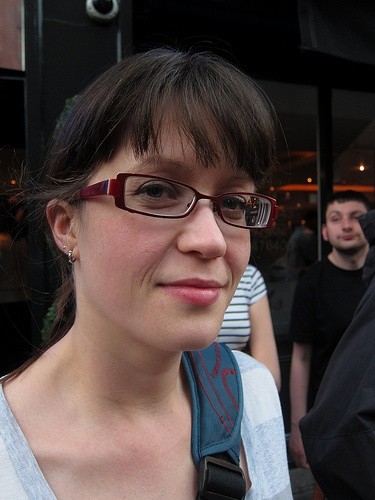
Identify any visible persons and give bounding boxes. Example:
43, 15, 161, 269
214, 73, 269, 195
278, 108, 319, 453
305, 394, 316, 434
0, 49, 294, 500
1, 189, 375, 500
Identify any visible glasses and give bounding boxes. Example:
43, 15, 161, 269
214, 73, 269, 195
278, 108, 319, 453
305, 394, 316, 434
70, 173, 277, 228
